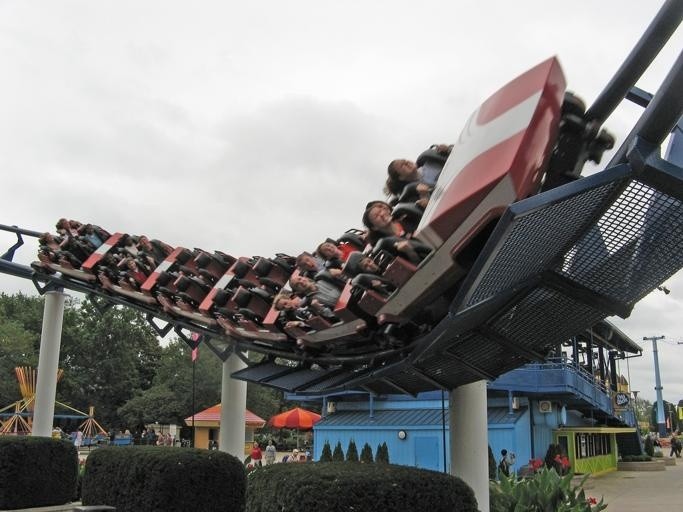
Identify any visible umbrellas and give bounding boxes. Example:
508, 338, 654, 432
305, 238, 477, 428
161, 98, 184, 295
269, 407, 322, 449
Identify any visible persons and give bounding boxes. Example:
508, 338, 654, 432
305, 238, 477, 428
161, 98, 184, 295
73, 427, 171, 451
545, 443, 570, 474
252, 442, 262, 467
274, 143, 451, 331
500, 450, 516, 476
654, 433, 682, 457
38, 218, 170, 292
286, 449, 298, 463
264, 439, 276, 466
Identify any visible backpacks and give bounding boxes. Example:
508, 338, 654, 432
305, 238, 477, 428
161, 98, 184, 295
498, 457, 509, 477
282, 455, 289, 462
